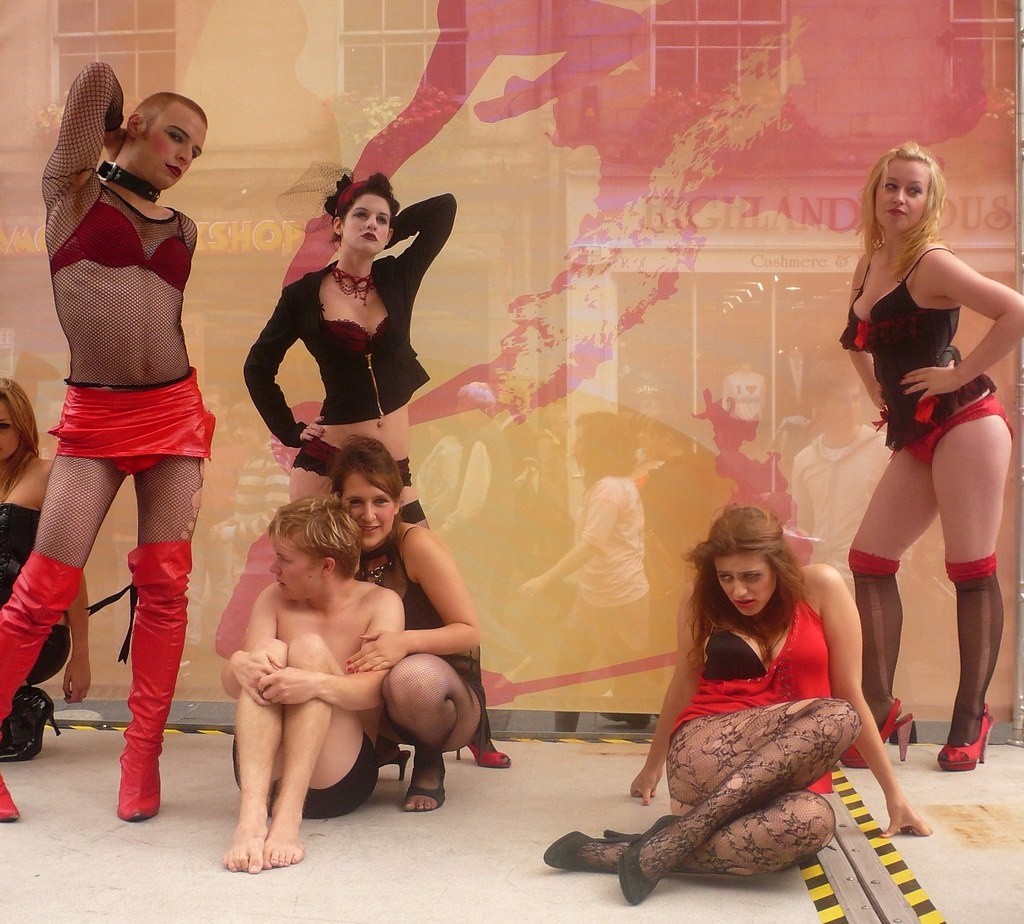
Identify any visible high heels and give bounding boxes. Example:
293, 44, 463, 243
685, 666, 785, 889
0, 686, 61, 762
937, 704, 992, 771
378, 749, 411, 781
841, 698, 913, 768
618, 815, 684, 905
403, 755, 445, 812
455, 740, 512, 768
544, 829, 641, 880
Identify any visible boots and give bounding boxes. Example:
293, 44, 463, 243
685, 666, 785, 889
117, 541, 193, 821
0, 550, 84, 822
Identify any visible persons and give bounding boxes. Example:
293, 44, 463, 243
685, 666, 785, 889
837, 139, 1024, 773
765, 347, 818, 432
119, 362, 919, 734
223, 431, 483, 812
224, 494, 405, 875
0, 61, 219, 822
245, 162, 512, 767
0, 378, 92, 762
723, 359, 766, 424
544, 507, 933, 905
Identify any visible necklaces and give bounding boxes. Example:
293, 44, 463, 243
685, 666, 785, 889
97, 160, 161, 203
330, 265, 377, 307
360, 560, 396, 585
